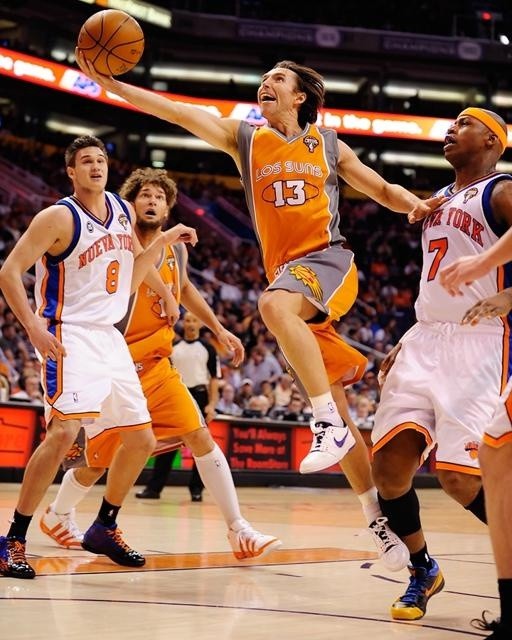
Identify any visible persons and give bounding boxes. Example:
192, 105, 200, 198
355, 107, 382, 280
469, 373, 512, 640
0, 136, 158, 579
371, 106, 512, 640
135, 311, 222, 502
73, 44, 450, 571
39, 165, 284, 560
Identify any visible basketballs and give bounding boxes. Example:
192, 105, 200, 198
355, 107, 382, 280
78, 10, 144, 76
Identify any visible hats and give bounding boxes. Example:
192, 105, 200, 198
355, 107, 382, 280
240, 378, 253, 387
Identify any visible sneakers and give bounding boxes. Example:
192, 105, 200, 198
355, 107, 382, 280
297, 415, 358, 476
224, 518, 284, 564
39, 504, 85, 551
135, 488, 161, 500
191, 491, 203, 502
470, 609, 512, 640
389, 557, 446, 622
0, 534, 36, 580
366, 516, 411, 572
81, 520, 145, 568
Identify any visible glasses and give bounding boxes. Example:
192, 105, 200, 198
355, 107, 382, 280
368, 376, 377, 380
360, 387, 372, 392
